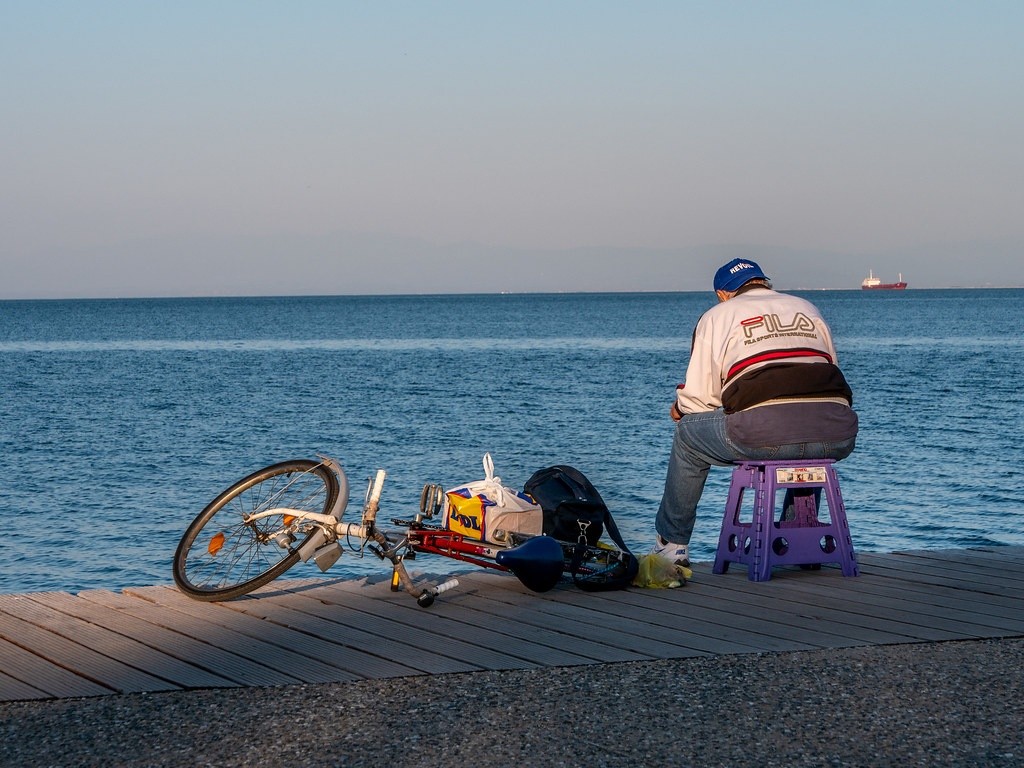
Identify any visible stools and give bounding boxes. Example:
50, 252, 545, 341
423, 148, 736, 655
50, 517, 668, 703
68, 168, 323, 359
712, 459, 861, 583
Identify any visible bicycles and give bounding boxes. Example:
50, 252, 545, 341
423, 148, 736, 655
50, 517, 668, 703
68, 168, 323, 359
172, 454, 610, 609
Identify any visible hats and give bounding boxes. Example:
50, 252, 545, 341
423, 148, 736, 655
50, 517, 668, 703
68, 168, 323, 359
712, 258, 770, 292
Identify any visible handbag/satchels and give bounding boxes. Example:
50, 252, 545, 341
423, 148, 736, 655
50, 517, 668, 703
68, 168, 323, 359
522, 464, 639, 591
442, 450, 541, 547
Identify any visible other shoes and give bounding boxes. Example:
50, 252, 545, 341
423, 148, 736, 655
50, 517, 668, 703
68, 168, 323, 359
646, 535, 713, 564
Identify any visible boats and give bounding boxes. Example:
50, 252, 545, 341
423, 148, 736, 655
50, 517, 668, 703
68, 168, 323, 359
861, 268, 908, 290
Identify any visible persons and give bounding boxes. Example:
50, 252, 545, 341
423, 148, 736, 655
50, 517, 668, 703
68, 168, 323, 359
645, 258, 859, 566
